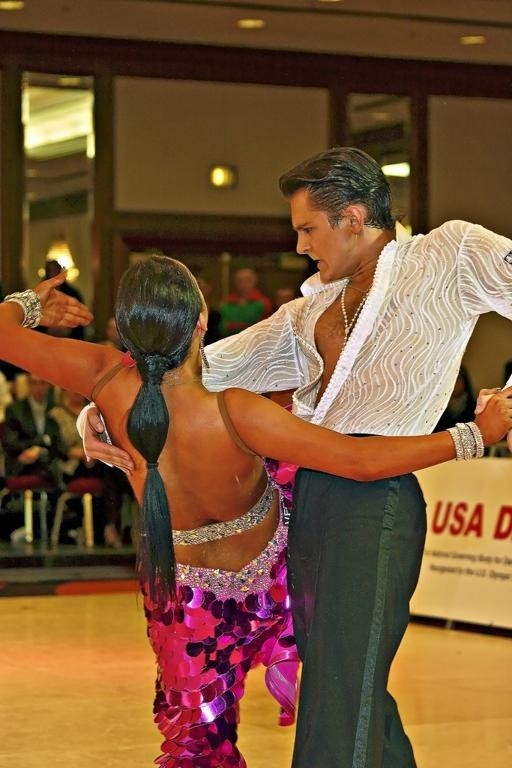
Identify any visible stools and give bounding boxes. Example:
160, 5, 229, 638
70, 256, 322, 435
4, 469, 107, 552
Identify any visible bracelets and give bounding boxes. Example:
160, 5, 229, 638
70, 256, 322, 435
445, 421, 484, 463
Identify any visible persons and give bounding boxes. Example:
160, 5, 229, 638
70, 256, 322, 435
435, 362, 477, 433
0, 261, 137, 548
0, 255, 512, 768
193, 262, 296, 346
76, 147, 512, 768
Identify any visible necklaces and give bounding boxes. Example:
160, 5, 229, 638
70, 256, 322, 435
340, 275, 374, 351
